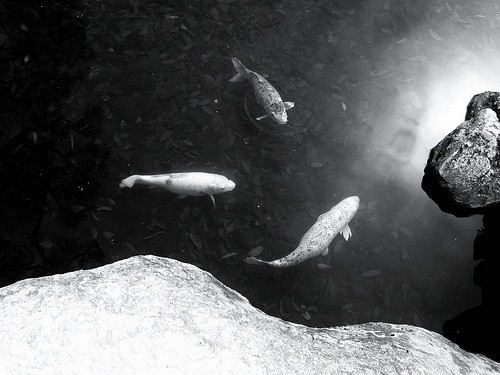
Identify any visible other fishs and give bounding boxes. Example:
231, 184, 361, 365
243, 194, 360, 268
227, 56, 295, 126
119, 170, 237, 208
